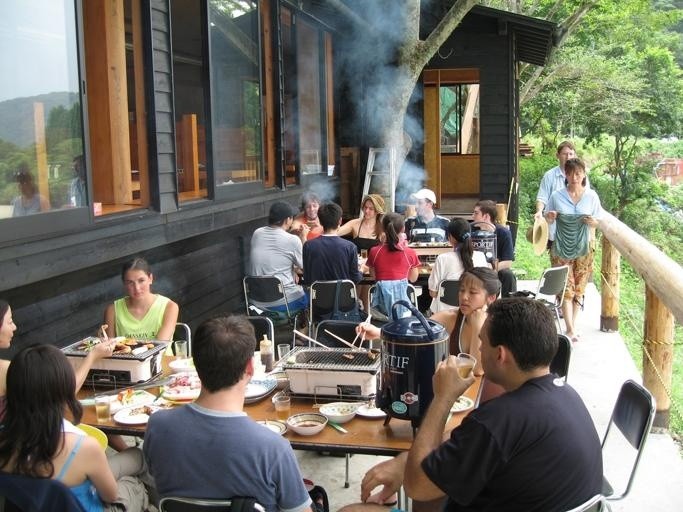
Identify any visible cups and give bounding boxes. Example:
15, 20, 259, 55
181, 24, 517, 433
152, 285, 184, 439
361, 249, 367, 258
175, 339, 187, 359
456, 353, 477, 378
275, 396, 290, 421
95, 396, 111, 423
277, 344, 290, 360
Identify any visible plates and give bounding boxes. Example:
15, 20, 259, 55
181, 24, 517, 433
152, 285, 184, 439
357, 403, 388, 418
254, 419, 287, 436
168, 358, 198, 372
159, 372, 201, 403
449, 395, 474, 413
107, 389, 156, 415
557, 214, 587, 223
243, 372, 278, 405
114, 405, 163, 425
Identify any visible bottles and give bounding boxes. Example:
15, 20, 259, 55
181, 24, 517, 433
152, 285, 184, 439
260, 334, 273, 371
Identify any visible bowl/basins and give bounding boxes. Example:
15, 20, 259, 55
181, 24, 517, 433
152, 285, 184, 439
287, 412, 329, 436
319, 401, 358, 424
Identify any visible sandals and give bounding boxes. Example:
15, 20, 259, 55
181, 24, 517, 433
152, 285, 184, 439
565, 332, 578, 342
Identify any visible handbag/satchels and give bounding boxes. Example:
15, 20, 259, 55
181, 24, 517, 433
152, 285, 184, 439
332, 279, 361, 321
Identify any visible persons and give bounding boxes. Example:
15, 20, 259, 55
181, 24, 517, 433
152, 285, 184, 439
428, 217, 490, 319
12, 170, 42, 217
545, 158, 602, 342
533, 140, 592, 317
468, 200, 517, 299
355, 266, 502, 377
100, 257, 179, 453
142, 315, 328, 512
0, 299, 116, 424
331, 297, 602, 512
288, 190, 324, 241
403, 188, 452, 315
249, 201, 311, 348
365, 211, 422, 320
335, 194, 389, 313
70, 154, 88, 206
302, 202, 369, 349
0, 344, 155, 512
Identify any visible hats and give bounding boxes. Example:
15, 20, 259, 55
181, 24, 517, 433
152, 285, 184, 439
361, 193, 387, 215
269, 202, 299, 220
526, 217, 549, 257
410, 189, 437, 205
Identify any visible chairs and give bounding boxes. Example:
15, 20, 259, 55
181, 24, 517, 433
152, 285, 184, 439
0, 473, 87, 512
159, 495, 265, 512
531, 264, 570, 335
366, 284, 418, 346
243, 277, 308, 349
308, 280, 360, 346
173, 322, 190, 357
314, 320, 372, 347
249, 317, 275, 350
598, 379, 654, 502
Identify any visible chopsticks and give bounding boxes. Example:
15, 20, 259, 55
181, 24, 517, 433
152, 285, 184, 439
351, 314, 372, 350
101, 326, 114, 349
312, 403, 368, 409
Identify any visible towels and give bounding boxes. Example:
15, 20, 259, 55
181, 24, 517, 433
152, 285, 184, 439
554, 213, 589, 261
